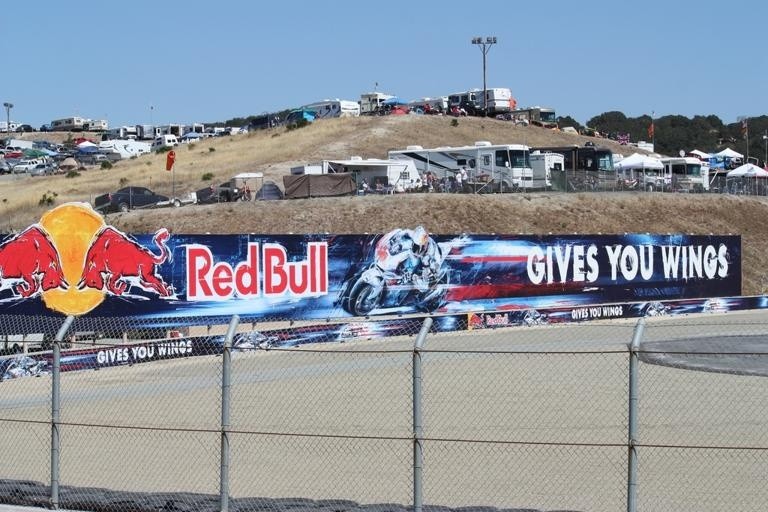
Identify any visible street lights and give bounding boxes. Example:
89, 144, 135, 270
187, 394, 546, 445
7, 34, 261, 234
471, 35, 497, 117
2, 102, 13, 134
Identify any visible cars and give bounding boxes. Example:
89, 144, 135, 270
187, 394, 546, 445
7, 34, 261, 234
0, 112, 250, 215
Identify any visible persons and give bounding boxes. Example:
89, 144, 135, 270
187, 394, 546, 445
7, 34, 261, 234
239, 180, 250, 202
449, 105, 468, 117
424, 103, 431, 114
386, 226, 444, 305
355, 165, 597, 194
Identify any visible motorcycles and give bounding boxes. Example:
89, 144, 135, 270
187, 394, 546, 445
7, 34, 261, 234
337, 322, 379, 340
343, 226, 451, 318
0, 356, 53, 382
225, 330, 278, 352
516, 309, 548, 327
642, 301, 668, 317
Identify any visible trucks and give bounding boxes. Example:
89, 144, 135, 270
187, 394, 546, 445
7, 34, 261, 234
0, 331, 50, 358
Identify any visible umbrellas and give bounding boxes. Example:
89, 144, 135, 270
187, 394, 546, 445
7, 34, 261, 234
408, 97, 435, 112
710, 148, 744, 159
382, 97, 407, 114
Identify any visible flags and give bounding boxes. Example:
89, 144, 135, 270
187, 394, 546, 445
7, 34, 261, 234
740, 120, 747, 140
647, 125, 653, 139
